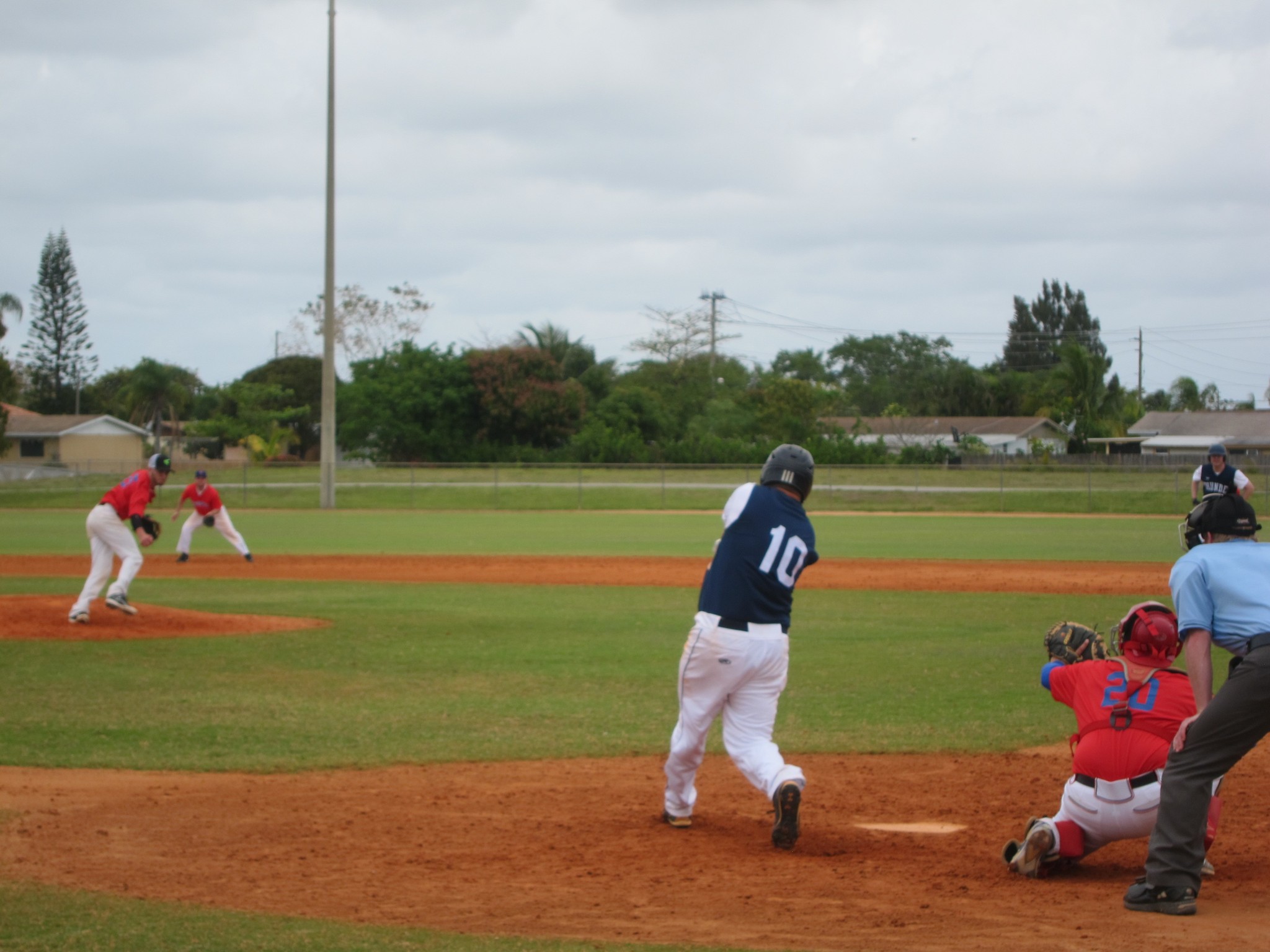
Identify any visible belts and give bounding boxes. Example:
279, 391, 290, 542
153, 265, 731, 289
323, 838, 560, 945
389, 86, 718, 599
1074, 772, 1158, 789
1248, 632, 1270, 649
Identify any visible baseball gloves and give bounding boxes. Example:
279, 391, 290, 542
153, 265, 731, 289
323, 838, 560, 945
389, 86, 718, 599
202, 516, 217, 529
1047, 620, 1108, 664
141, 516, 161, 540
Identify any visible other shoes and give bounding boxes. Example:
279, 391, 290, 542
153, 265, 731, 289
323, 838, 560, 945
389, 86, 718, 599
177, 553, 188, 562
245, 554, 253, 562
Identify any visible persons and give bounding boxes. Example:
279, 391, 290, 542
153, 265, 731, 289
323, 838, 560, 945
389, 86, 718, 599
1003, 618, 1212, 875
1121, 491, 1270, 914
1189, 443, 1254, 503
67, 452, 172, 627
662, 444, 822, 851
172, 468, 254, 563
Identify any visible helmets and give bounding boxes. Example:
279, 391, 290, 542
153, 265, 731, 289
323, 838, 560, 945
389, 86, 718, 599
1208, 444, 1227, 457
1179, 494, 1261, 551
1111, 600, 1183, 668
760, 444, 814, 501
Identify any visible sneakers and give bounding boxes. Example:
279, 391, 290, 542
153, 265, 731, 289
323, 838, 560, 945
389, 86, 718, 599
1123, 875, 1197, 914
771, 781, 800, 847
105, 594, 138, 615
667, 813, 692, 826
69, 611, 88, 623
1008, 823, 1054, 878
1201, 860, 1215, 876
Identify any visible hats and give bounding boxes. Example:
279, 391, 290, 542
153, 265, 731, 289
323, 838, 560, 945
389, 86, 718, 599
148, 453, 174, 473
196, 470, 207, 477
